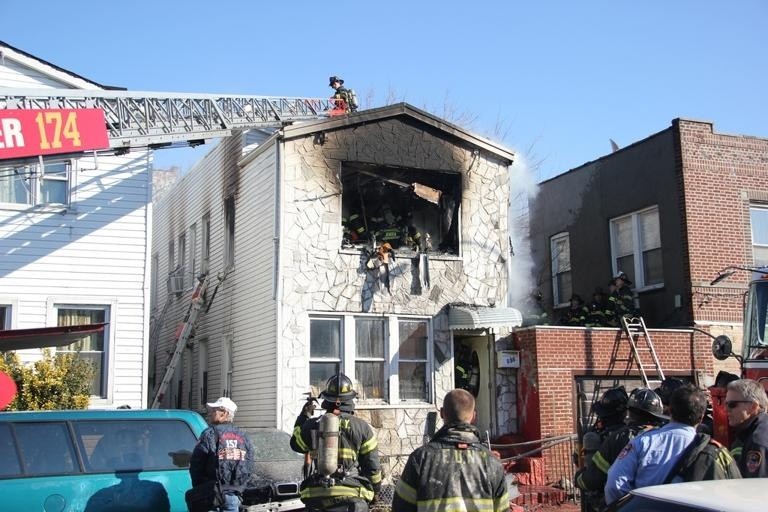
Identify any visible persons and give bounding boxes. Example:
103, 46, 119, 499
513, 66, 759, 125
185, 393, 258, 512
343, 194, 423, 253
288, 372, 383, 511
89, 404, 157, 470
328, 75, 360, 116
527, 269, 639, 328
565, 374, 768, 510
389, 386, 514, 511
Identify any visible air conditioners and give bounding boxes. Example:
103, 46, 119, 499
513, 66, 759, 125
166, 276, 183, 294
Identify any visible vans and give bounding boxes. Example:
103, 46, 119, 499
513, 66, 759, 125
0, 409, 211, 512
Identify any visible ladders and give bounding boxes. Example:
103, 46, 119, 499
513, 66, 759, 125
586, 326, 639, 428
150, 274, 209, 409
621, 316, 666, 391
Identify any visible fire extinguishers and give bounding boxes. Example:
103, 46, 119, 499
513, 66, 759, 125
319, 414, 339, 486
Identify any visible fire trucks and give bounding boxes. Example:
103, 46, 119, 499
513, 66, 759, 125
708, 264, 767, 404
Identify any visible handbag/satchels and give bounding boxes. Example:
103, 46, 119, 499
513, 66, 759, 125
186, 479, 226, 511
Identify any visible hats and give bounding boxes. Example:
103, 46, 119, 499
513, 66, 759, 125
329, 76, 344, 86
207, 396, 237, 411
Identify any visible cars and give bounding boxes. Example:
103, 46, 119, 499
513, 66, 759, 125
599, 477, 768, 511
241, 427, 316, 511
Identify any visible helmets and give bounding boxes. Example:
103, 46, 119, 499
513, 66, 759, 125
612, 270, 632, 285
628, 388, 671, 420
321, 370, 357, 403
592, 287, 607, 296
654, 375, 688, 403
528, 289, 544, 299
592, 386, 627, 417
569, 294, 584, 305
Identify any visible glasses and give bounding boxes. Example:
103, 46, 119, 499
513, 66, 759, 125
725, 400, 752, 408
